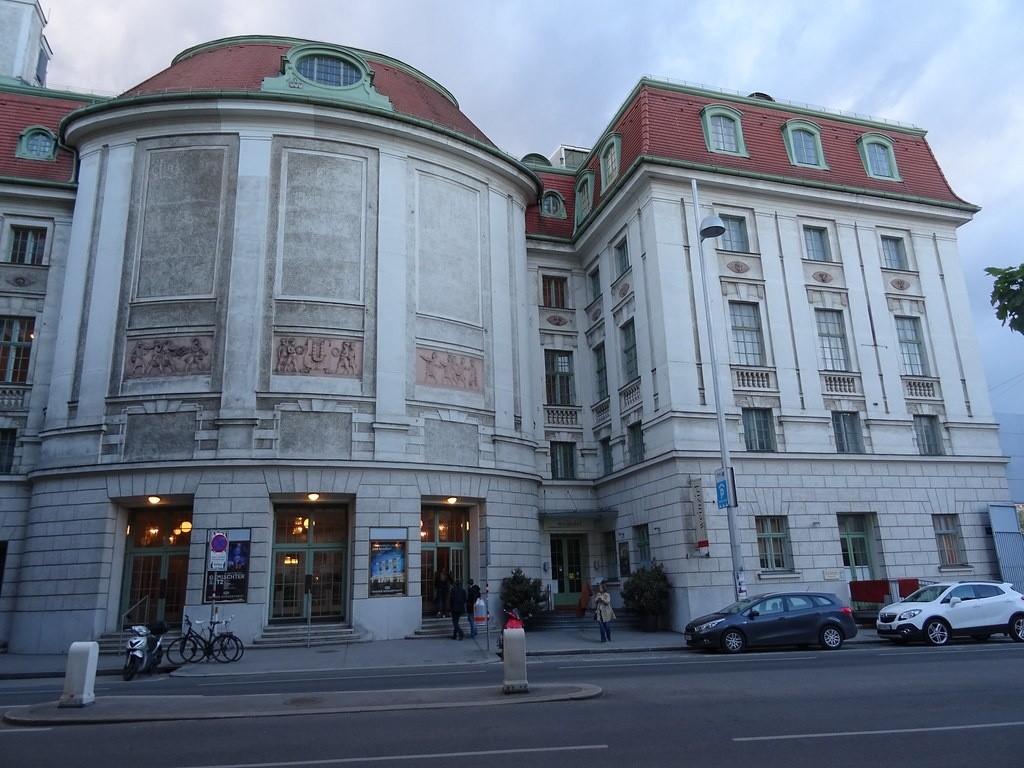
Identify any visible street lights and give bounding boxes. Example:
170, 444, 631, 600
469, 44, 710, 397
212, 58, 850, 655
688, 177, 749, 600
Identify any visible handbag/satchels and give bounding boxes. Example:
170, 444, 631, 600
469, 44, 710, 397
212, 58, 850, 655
593, 613, 597, 620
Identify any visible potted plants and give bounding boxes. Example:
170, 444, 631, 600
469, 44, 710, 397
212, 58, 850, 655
624, 567, 670, 632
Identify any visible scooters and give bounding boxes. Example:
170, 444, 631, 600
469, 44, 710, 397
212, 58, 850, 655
121, 625, 163, 681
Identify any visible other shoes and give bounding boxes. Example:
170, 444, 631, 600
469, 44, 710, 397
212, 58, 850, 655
452, 637, 456, 639
459, 634, 464, 640
442, 615, 445, 618
437, 612, 441, 617
601, 639, 606, 642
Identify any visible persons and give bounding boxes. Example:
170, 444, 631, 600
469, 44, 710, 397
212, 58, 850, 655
594, 583, 613, 642
449, 579, 481, 641
433, 567, 451, 618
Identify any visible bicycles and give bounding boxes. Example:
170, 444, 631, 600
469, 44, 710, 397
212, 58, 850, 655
165, 615, 244, 666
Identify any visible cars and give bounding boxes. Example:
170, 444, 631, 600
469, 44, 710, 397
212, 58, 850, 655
685, 589, 858, 653
875, 580, 1024, 646
